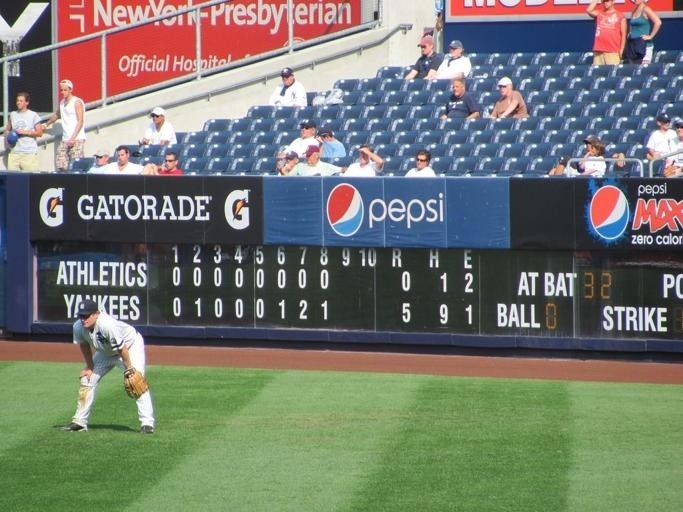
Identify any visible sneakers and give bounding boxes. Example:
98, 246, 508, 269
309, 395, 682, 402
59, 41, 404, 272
59, 422, 84, 433
139, 425, 154, 434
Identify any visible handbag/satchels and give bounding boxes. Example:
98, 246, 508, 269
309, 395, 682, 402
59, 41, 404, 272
627, 37, 646, 60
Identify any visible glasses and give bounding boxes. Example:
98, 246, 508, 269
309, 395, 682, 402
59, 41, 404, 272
165, 159, 174, 162
417, 159, 426, 162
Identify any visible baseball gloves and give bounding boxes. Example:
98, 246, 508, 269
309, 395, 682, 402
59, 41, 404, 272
124, 367, 148, 399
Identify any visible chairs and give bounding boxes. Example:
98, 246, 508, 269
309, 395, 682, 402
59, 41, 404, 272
34, 48, 683, 180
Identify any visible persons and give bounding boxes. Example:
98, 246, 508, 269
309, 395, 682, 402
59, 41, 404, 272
3, 78, 183, 176
404, 150, 436, 177
626, 0, 662, 65
547, 112, 683, 177
275, 120, 384, 177
54, 300, 155, 434
490, 77, 530, 119
269, 67, 307, 105
586, 0, 627, 65
404, 0, 481, 119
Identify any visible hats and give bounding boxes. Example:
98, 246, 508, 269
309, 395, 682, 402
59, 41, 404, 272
583, 136, 599, 145
657, 113, 670, 122
148, 107, 165, 119
417, 37, 434, 47
93, 150, 109, 157
447, 40, 464, 49
497, 76, 513, 87
285, 118, 320, 160
76, 300, 97, 315
281, 67, 293, 76
59, 80, 73, 88
